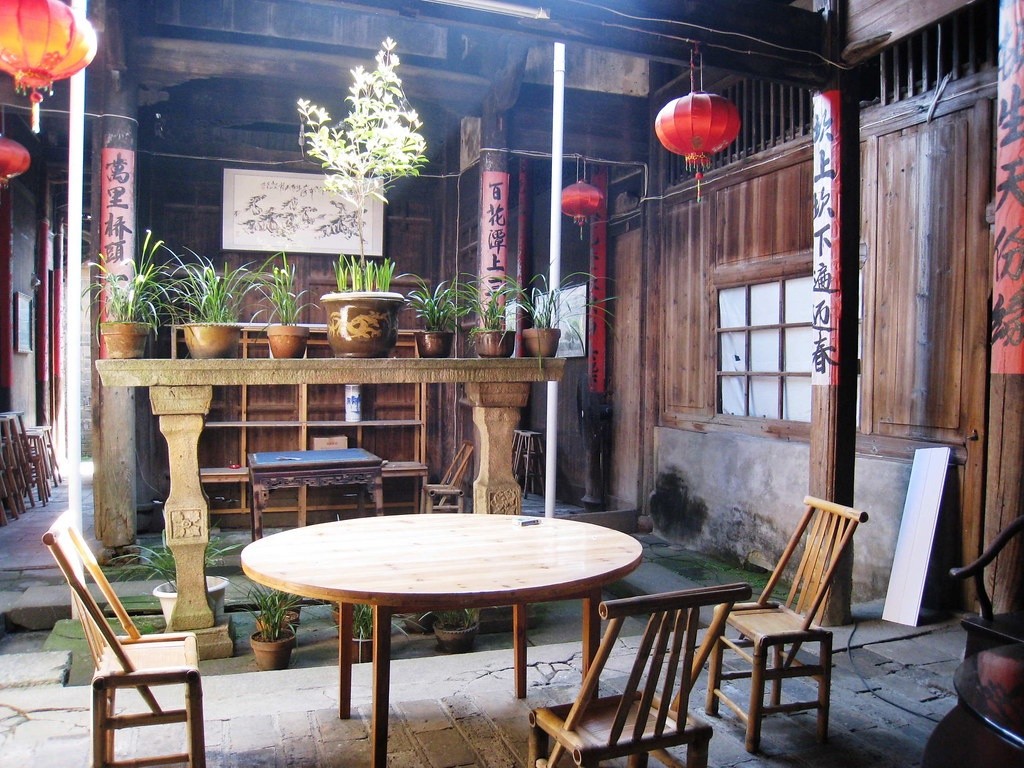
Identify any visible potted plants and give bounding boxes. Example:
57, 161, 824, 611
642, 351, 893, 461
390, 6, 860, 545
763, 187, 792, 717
166, 244, 284, 359
100, 509, 244, 625
392, 272, 476, 358
230, 576, 306, 646
453, 272, 528, 358
417, 606, 501, 654
78, 230, 189, 359
241, 575, 304, 615
219, 572, 314, 670
491, 256, 622, 372
296, 36, 431, 358
325, 603, 428, 664
244, 248, 323, 358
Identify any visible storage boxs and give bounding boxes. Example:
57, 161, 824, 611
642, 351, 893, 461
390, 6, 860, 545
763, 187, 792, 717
309, 434, 348, 450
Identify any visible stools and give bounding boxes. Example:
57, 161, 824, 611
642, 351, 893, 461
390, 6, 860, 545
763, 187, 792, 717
512, 430, 546, 499
0, 411, 62, 525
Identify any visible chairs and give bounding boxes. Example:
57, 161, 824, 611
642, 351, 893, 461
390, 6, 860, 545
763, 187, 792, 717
526, 583, 752, 768
420, 440, 475, 514
41, 507, 206, 768
705, 495, 868, 753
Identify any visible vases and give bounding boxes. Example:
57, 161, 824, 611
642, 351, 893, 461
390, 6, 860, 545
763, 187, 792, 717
345, 383, 362, 422
400, 610, 432, 634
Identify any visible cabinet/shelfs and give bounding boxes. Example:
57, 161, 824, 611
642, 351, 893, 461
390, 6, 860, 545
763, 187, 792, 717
164, 324, 430, 532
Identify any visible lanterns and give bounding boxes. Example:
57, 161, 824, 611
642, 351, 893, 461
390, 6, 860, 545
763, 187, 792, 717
561, 157, 605, 241
0, 136, 30, 189
654, 46, 741, 202
0, 0, 98, 132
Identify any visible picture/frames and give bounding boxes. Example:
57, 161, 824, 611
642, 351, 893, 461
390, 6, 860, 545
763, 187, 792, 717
16, 291, 34, 354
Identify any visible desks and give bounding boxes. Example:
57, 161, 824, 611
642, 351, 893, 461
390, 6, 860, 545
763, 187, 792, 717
247, 446, 384, 543
239, 512, 644, 768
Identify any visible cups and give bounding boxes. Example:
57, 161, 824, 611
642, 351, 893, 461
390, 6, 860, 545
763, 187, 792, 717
344, 384, 362, 423
